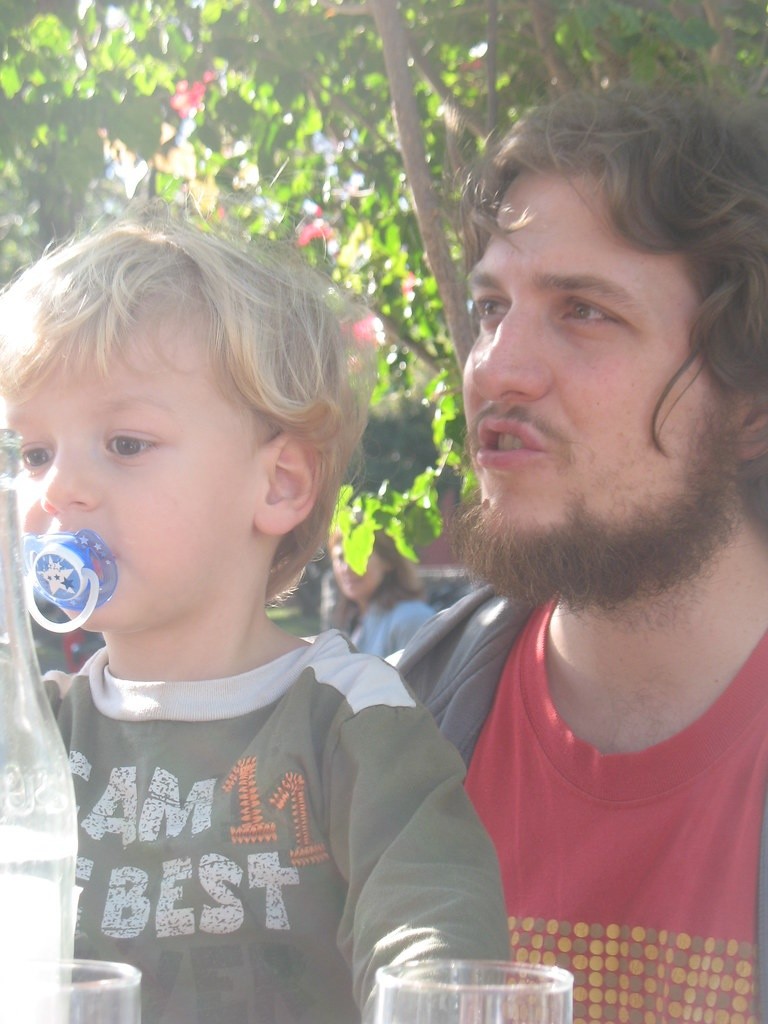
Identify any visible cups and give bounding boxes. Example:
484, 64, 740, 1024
374, 958, 575, 1024
69, 959, 143, 1024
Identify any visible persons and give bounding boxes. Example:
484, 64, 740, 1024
383, 80, 768, 1024
331, 509, 435, 658
1, 209, 508, 1023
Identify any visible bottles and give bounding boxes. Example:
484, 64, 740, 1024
0, 429, 80, 1024
